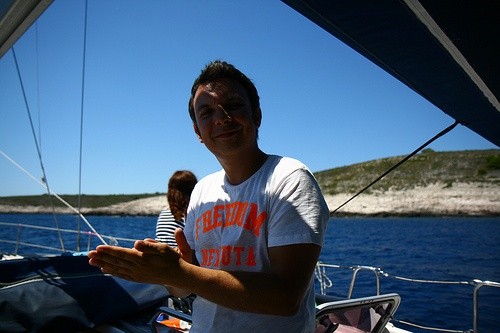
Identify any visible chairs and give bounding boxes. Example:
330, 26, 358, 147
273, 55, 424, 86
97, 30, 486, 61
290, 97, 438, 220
315, 293, 401, 332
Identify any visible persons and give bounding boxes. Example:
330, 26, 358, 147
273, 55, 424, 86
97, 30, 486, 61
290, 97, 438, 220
156, 170, 198, 316
87, 59, 330, 333
